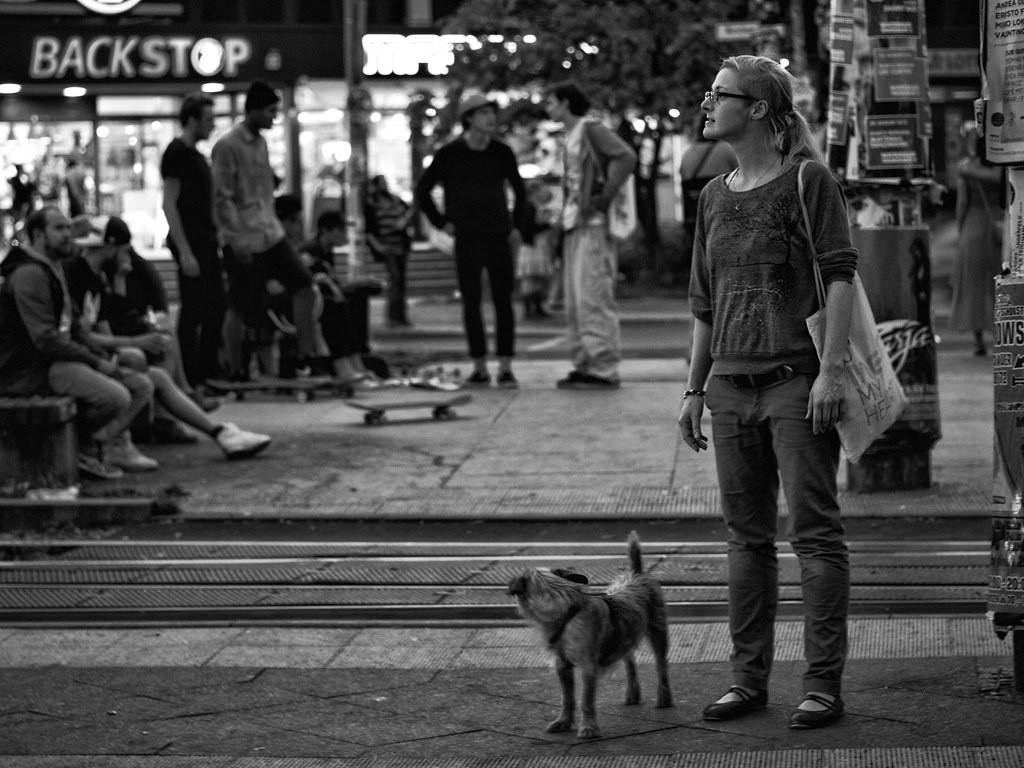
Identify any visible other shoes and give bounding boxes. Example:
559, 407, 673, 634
974, 346, 988, 358
197, 354, 381, 378
189, 394, 219, 413
145, 418, 202, 444
789, 694, 845, 730
703, 687, 770, 721
461, 370, 519, 388
557, 371, 622, 390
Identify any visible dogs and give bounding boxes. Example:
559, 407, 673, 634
505, 529, 674, 738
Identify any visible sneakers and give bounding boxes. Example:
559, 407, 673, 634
101, 441, 157, 469
216, 421, 273, 460
75, 448, 123, 481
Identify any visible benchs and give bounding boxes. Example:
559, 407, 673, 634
0, 394, 85, 486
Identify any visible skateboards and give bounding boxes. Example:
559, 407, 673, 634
205, 375, 368, 404
385, 366, 462, 392
342, 394, 473, 426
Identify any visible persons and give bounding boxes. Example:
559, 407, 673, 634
414, 93, 540, 390
544, 81, 637, 391
0, 78, 397, 481
676, 53, 860, 728
363, 176, 419, 326
680, 114, 739, 249
949, 130, 1010, 356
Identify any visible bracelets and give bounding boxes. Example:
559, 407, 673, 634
683, 390, 706, 399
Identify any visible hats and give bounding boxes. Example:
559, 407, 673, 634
319, 209, 354, 229
102, 215, 133, 243
459, 94, 501, 128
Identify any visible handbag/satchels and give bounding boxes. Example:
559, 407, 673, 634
805, 267, 909, 467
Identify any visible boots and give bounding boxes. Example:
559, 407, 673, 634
520, 289, 554, 322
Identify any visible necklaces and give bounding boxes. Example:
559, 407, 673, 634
732, 153, 781, 212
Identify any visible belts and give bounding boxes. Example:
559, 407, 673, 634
729, 363, 800, 389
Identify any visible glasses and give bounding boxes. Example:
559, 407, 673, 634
705, 90, 758, 103
